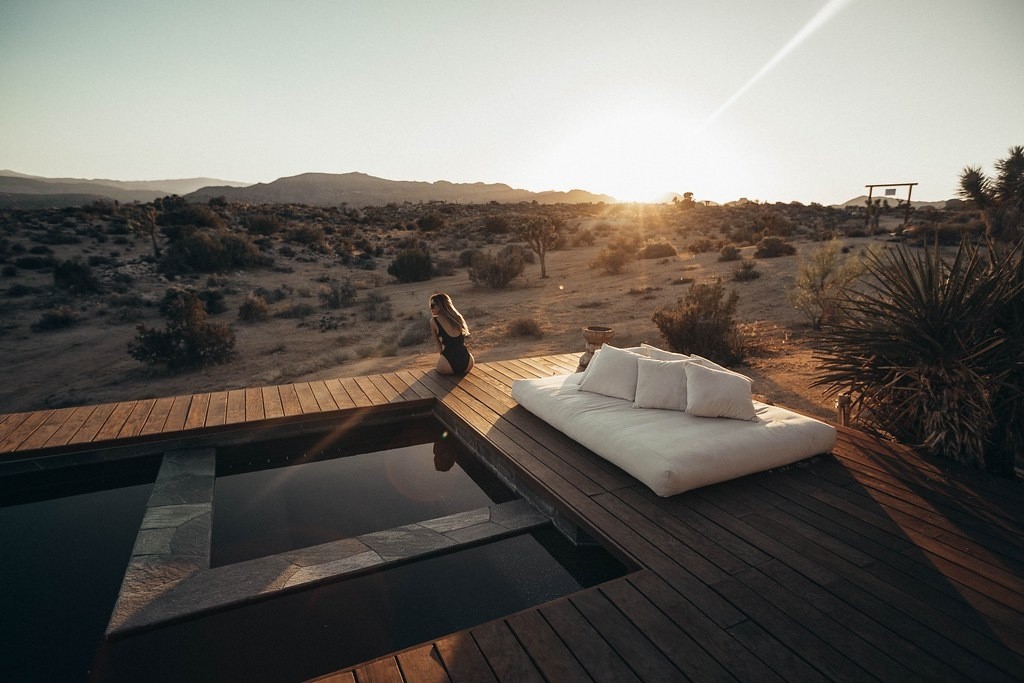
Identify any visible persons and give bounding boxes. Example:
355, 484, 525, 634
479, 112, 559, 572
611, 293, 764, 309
428, 293, 474, 374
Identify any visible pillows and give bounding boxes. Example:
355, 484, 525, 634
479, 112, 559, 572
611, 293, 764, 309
578, 343, 756, 420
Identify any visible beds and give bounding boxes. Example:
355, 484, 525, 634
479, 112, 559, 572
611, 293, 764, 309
511, 369, 839, 497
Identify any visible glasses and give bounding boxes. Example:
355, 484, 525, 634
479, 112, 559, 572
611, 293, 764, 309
431, 303, 435, 309
